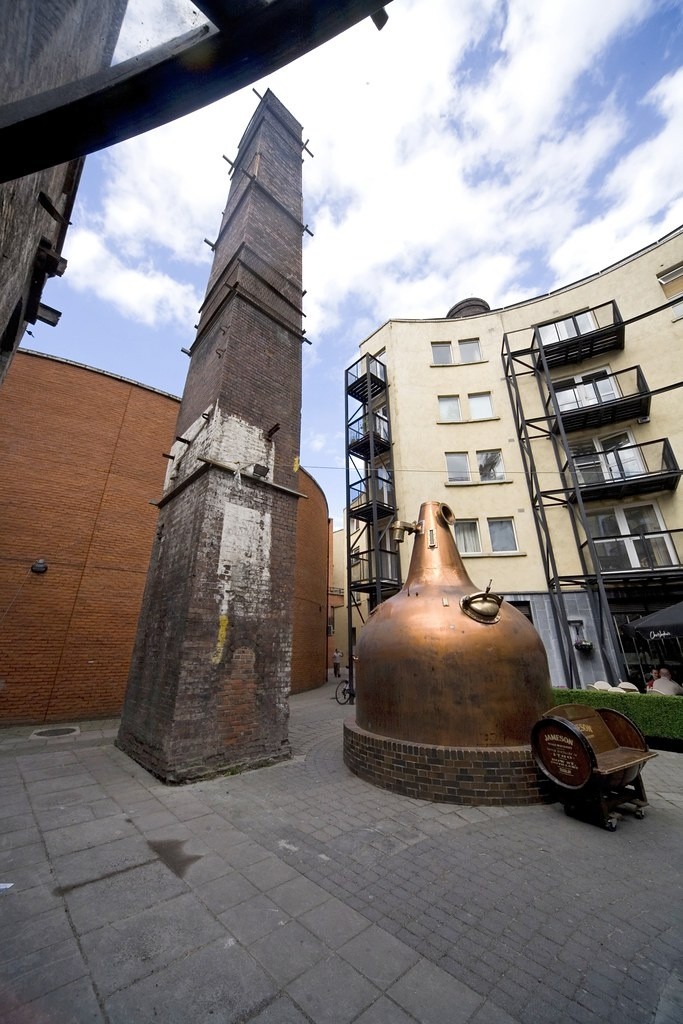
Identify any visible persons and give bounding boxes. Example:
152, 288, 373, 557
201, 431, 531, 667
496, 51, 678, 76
332, 648, 343, 678
639, 647, 651, 673
646, 668, 683, 697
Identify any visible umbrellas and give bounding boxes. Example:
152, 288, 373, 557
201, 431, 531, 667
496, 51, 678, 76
623, 601, 683, 641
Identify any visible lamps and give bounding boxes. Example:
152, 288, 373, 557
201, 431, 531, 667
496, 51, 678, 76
252, 463, 270, 478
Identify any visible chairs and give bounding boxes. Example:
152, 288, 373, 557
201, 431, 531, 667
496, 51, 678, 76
586, 681, 666, 696
530, 703, 660, 833
